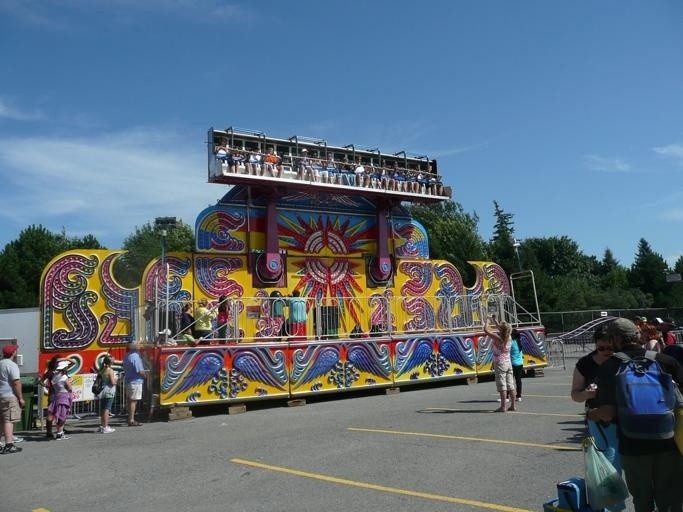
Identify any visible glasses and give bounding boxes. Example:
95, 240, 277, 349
596, 345, 613, 352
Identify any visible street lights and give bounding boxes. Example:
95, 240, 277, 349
153, 215, 177, 264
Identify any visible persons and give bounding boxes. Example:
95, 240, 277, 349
177, 298, 216, 346
0, 345, 25, 454
483, 314, 516, 412
97, 356, 117, 434
214, 137, 444, 196
287, 290, 307, 341
571, 316, 683, 512
48, 361, 72, 440
217, 295, 232, 343
490, 329, 523, 402
37, 354, 70, 438
122, 343, 146, 426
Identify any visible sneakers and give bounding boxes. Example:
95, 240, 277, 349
0, 426, 118, 455
493, 396, 522, 413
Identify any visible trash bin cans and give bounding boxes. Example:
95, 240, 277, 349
13, 377, 35, 432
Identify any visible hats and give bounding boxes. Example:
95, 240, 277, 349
54, 360, 71, 370
631, 312, 680, 329
1, 344, 19, 356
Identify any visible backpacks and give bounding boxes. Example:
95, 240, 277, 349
610, 349, 676, 441
90, 372, 103, 396
